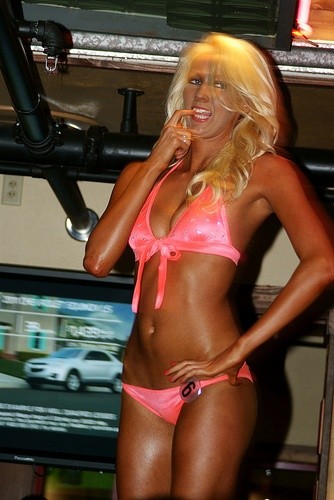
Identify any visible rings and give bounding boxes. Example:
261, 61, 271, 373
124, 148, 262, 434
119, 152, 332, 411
182, 134, 186, 144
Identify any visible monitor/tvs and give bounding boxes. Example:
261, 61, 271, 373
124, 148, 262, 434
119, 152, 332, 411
1, 263, 135, 472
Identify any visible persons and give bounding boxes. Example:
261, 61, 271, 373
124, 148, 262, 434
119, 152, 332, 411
84, 31, 330, 500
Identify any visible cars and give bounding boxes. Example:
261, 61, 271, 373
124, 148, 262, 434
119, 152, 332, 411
24, 346, 125, 394
